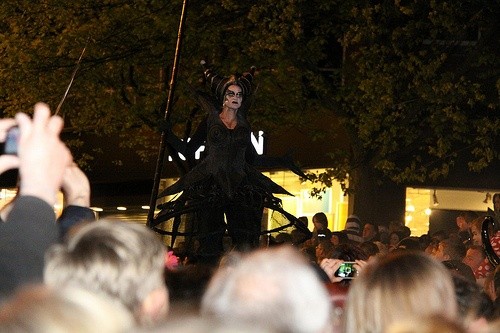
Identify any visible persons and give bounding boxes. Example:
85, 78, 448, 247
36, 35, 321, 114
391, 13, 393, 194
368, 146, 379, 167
164, 60, 270, 262
0, 105, 495, 333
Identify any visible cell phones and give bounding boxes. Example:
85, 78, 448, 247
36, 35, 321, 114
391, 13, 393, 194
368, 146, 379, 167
3, 126, 20, 155
334, 262, 360, 280
317, 233, 325, 237
